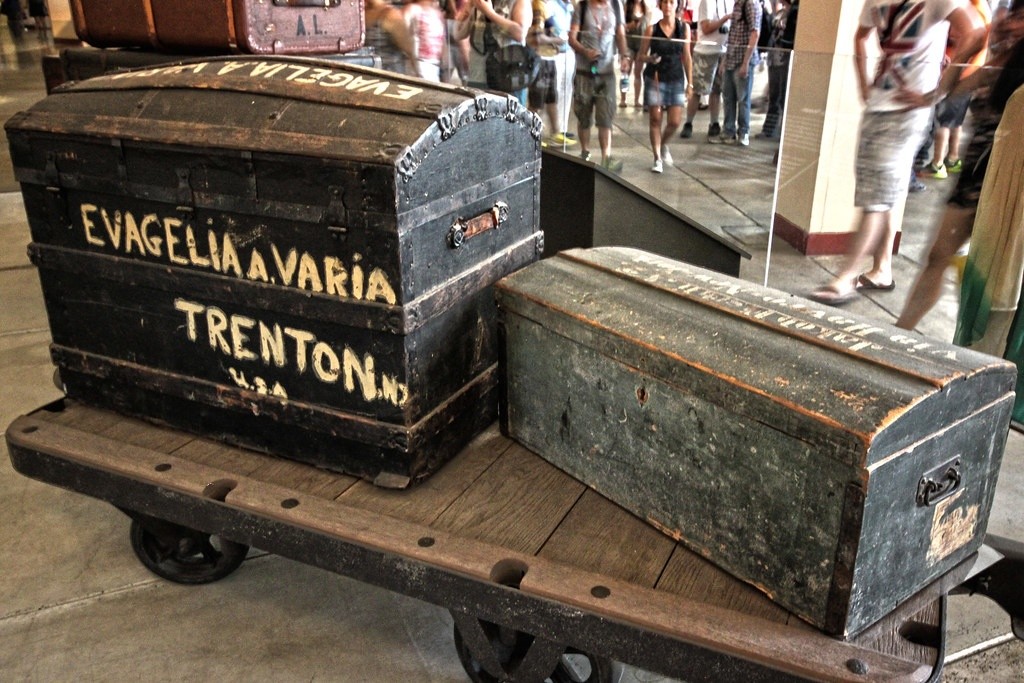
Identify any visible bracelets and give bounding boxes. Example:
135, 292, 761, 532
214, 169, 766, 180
688, 84, 693, 88
923, 87, 946, 105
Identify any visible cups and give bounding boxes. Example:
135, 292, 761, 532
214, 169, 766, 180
590, 59, 599, 78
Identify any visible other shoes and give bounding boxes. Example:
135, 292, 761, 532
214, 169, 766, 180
908, 181, 926, 191
619, 102, 627, 107
602, 158, 622, 172
661, 143, 673, 166
681, 121, 693, 137
547, 133, 576, 147
708, 123, 720, 135
635, 103, 643, 107
581, 150, 590, 160
651, 159, 663, 172
755, 130, 778, 139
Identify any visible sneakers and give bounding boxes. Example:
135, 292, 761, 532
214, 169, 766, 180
708, 131, 736, 144
738, 132, 749, 145
915, 162, 948, 178
944, 157, 962, 172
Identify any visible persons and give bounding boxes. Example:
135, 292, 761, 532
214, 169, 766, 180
365, 0, 800, 173
807, 0, 1024, 331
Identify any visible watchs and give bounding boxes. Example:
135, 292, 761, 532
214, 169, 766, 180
621, 57, 630, 61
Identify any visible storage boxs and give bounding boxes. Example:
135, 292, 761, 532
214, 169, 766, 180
493, 244, 1017, 642
4, 53, 549, 491
69, 0, 367, 56
41, 46, 385, 95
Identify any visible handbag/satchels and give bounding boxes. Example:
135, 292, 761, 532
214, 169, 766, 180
485, 42, 531, 90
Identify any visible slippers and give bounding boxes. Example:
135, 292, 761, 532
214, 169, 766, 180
810, 285, 860, 304
852, 274, 895, 290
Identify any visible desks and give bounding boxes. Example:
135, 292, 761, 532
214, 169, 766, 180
0, 398, 950, 683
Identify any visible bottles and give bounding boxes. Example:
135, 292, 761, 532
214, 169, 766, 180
621, 71, 629, 92
